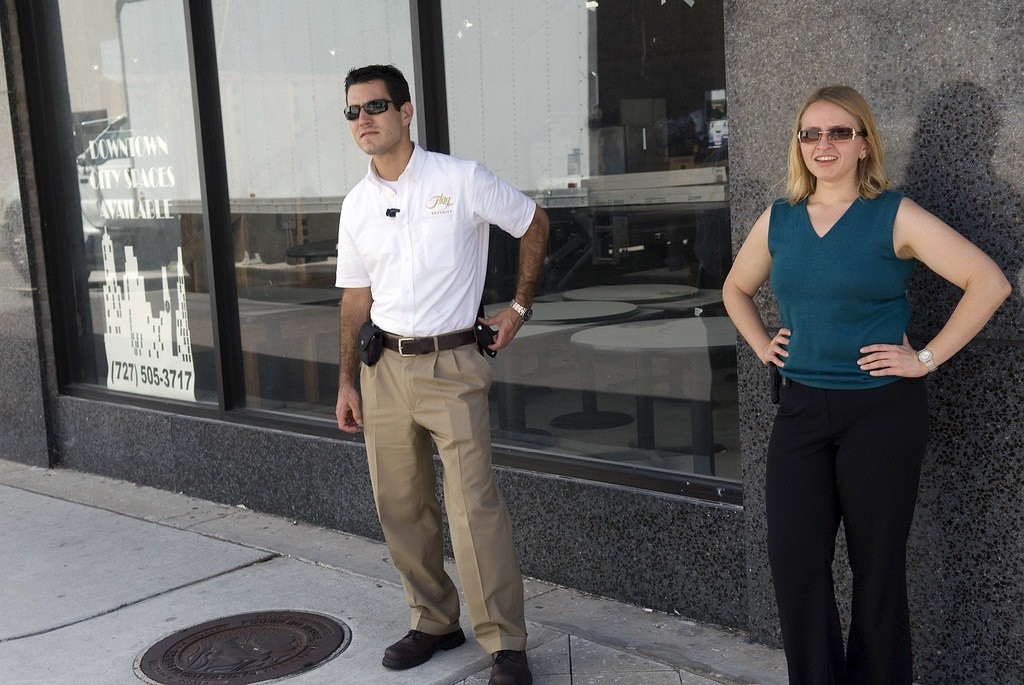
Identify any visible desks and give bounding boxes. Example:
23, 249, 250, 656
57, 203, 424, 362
88, 257, 736, 478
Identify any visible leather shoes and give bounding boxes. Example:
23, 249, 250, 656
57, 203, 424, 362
382, 626, 466, 669
488, 648, 532, 685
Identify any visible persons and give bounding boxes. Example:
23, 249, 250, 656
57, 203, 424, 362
335, 66, 549, 685
721, 85, 1013, 685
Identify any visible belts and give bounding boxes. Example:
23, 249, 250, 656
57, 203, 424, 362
381, 329, 477, 357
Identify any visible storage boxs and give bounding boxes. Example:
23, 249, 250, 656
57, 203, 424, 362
669, 156, 697, 170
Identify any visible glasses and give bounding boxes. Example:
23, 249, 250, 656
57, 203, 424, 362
797, 128, 868, 143
343, 99, 402, 121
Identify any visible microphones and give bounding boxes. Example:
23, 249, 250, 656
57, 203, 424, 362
386, 208, 400, 217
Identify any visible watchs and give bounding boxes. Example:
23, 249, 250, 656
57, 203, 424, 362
508, 298, 534, 323
918, 348, 938, 376
